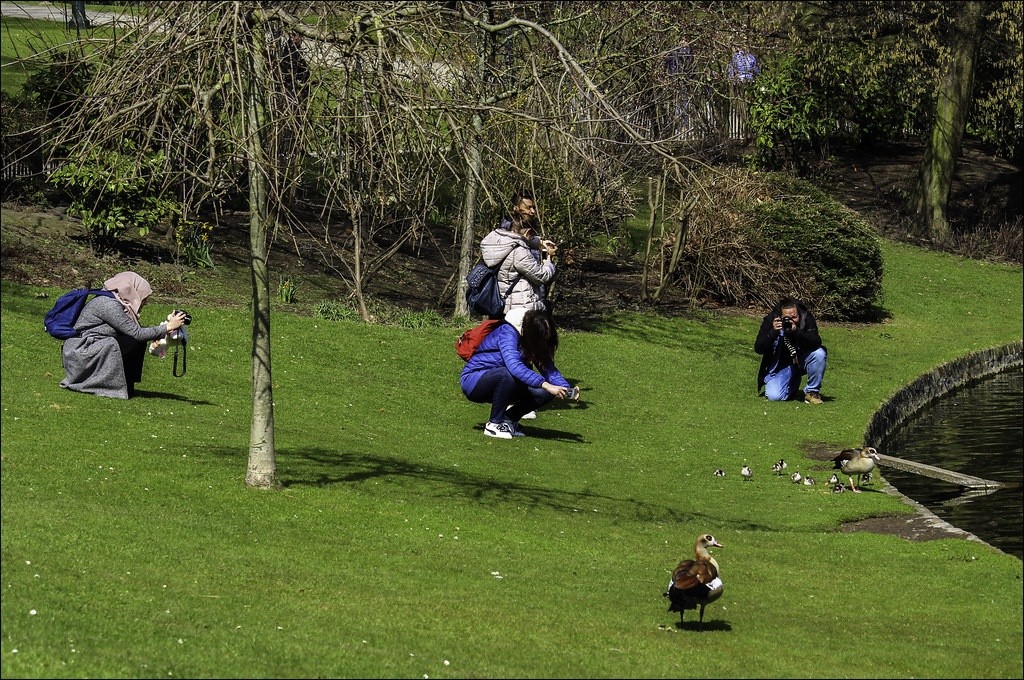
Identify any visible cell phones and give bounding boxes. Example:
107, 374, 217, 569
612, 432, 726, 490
542, 249, 548, 261
562, 388, 578, 400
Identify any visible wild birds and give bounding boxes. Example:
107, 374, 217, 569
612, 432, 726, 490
713, 447, 881, 493
663, 534, 724, 625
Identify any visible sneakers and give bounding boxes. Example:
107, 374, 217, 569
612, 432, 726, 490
502, 419, 525, 436
805, 393, 824, 405
484, 422, 513, 439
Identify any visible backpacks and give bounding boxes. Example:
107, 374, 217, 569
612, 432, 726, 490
465, 243, 527, 316
44, 287, 114, 340
455, 320, 522, 361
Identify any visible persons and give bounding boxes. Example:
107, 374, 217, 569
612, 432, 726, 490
480, 195, 558, 372
460, 307, 579, 439
754, 297, 827, 405
59, 271, 187, 401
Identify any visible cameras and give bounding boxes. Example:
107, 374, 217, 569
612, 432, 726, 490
174, 309, 192, 326
775, 316, 794, 332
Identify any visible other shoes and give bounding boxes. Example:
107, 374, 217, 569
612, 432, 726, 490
506, 405, 536, 419
